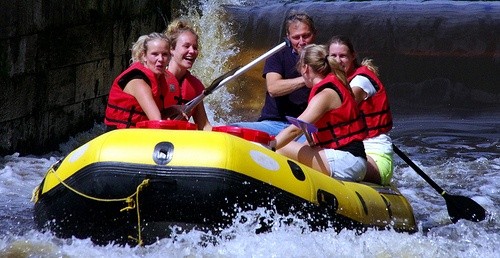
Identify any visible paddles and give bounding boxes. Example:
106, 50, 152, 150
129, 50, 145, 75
170, 65, 240, 121
392, 144, 489, 224
183, 36, 291, 111
285, 116, 319, 148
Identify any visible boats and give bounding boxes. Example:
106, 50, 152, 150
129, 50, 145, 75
33, 118, 418, 249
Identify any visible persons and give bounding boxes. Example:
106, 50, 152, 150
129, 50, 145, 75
162, 19, 214, 133
104, 33, 181, 132
267, 43, 368, 183
230, 12, 316, 137
325, 35, 395, 185
